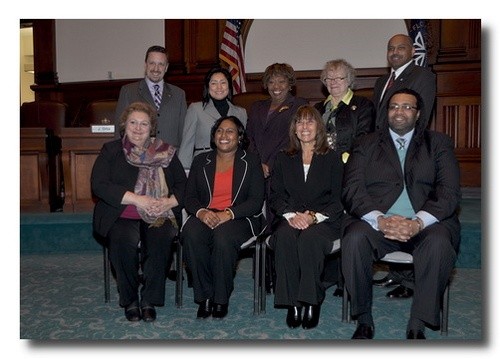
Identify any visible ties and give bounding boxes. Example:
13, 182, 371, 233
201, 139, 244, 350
153, 85, 163, 111
396, 137, 409, 151
379, 72, 397, 108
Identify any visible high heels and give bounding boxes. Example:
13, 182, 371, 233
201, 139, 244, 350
286, 303, 302, 328
303, 303, 322, 330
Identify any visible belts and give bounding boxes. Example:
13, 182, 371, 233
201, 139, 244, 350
195, 146, 211, 152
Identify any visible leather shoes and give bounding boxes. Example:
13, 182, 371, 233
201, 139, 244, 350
168, 269, 185, 282
386, 284, 414, 299
141, 307, 159, 321
265, 278, 276, 295
124, 299, 140, 321
372, 274, 402, 288
405, 328, 426, 339
196, 297, 213, 319
212, 302, 231, 320
350, 324, 376, 341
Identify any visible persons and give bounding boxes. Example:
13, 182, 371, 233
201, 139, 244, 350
247, 63, 309, 294
264, 105, 349, 330
185, 115, 265, 320
312, 59, 373, 298
178, 67, 247, 178
341, 88, 462, 340
372, 34, 437, 299
115, 45, 187, 281
89, 102, 187, 323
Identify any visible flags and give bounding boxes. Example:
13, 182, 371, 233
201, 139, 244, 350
219, 19, 246, 95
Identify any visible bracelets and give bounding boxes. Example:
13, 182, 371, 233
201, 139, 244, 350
415, 219, 422, 232
310, 211, 318, 225
226, 210, 233, 218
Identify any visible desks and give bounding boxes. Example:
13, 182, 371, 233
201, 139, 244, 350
20, 127, 59, 213
58, 129, 116, 213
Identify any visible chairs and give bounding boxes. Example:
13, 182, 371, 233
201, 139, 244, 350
20, 101, 66, 137
232, 92, 270, 112
91, 170, 454, 335
91, 100, 118, 126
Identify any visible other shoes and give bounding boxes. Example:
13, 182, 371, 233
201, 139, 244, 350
334, 285, 350, 298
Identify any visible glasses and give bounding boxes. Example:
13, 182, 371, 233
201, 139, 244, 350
323, 77, 345, 84
389, 104, 418, 113
